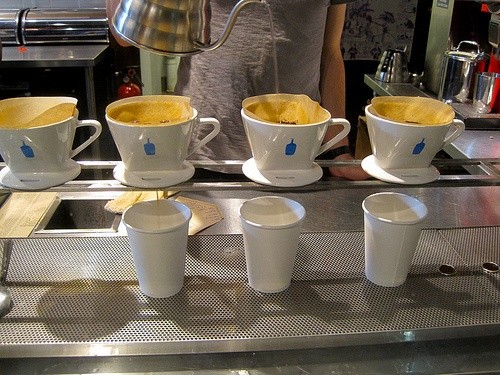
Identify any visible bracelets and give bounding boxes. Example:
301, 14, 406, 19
324, 145, 350, 160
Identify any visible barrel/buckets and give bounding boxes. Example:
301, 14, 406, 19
437, 40, 489, 105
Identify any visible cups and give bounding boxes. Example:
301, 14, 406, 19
241, 99, 351, 188
361, 192, 428, 286
472, 72, 500, 114
360, 103, 466, 185
0, 97, 101, 191
238, 196, 306, 293
105, 101, 221, 189
122, 199, 192, 299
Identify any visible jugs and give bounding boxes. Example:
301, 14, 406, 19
111, 0, 264, 57
375, 48, 410, 83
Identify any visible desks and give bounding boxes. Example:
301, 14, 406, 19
0, 44, 110, 180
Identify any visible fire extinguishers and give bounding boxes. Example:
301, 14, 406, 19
115, 66, 144, 100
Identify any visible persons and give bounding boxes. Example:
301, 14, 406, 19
106, 0, 370, 183
